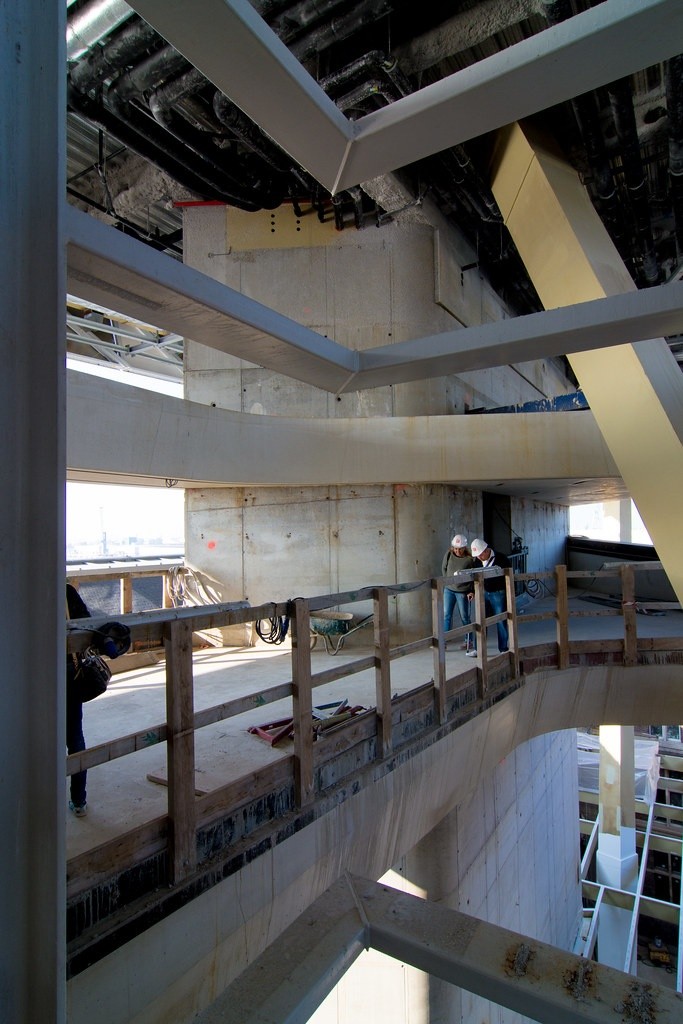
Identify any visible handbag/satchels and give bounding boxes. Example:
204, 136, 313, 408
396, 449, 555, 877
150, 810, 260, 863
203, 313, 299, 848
73, 646, 112, 704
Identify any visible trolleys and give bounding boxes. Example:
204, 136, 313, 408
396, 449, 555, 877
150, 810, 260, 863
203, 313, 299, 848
308, 610, 373, 656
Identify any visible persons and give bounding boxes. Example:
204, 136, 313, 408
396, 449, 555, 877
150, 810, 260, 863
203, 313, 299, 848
428, 534, 475, 651
465, 539, 511, 656
65, 585, 96, 817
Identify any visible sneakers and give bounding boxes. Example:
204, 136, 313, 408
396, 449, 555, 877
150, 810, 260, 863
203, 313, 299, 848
466, 651, 477, 657
460, 640, 474, 650
68, 798, 88, 817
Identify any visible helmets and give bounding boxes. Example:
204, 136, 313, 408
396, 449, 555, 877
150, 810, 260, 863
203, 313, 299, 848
451, 534, 467, 548
471, 539, 487, 557
